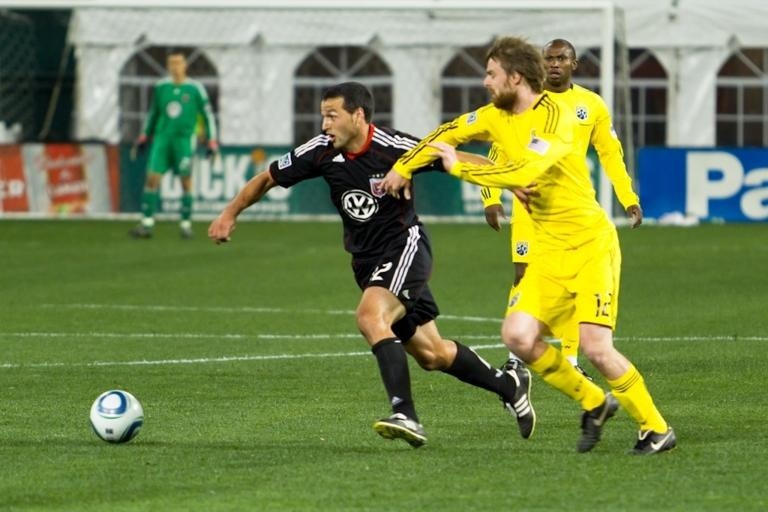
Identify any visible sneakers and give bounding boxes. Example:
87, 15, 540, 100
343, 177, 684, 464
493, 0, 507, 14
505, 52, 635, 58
626, 422, 677, 456
373, 410, 428, 450
574, 387, 621, 453
497, 355, 537, 442
127, 223, 154, 239
178, 225, 195, 240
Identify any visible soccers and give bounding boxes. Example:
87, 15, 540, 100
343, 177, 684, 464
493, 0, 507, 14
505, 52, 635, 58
89, 390, 144, 445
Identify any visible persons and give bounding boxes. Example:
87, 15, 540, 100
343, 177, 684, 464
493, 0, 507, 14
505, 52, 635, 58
128, 50, 220, 240
206, 79, 544, 449
377, 35, 677, 456
479, 38, 645, 383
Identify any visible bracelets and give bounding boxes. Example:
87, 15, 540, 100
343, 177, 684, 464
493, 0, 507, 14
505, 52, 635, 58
207, 139, 218, 150
138, 134, 147, 142
